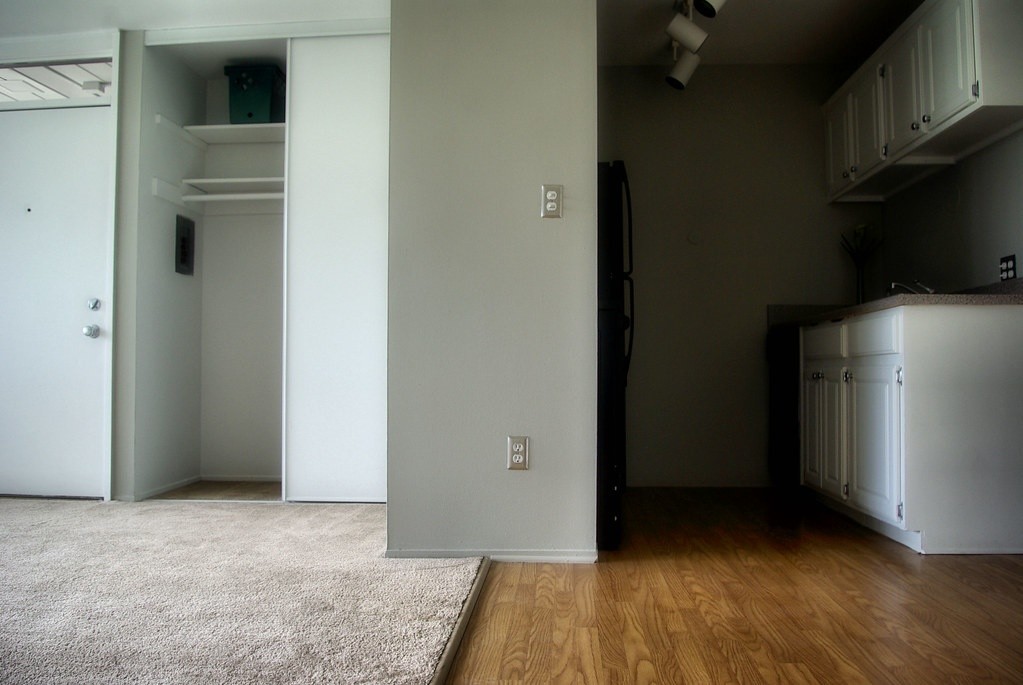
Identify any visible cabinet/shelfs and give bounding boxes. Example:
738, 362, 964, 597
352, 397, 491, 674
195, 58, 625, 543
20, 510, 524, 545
799, 304, 1022, 554
820, 0, 1023, 204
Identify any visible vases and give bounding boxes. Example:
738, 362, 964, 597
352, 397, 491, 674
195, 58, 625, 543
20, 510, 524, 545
856, 270, 865, 305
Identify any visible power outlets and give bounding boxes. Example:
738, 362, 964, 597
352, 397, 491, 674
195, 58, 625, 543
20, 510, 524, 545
506, 435, 530, 471
999, 254, 1016, 281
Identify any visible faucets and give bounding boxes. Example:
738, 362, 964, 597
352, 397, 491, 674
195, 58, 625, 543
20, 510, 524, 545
891, 279, 934, 294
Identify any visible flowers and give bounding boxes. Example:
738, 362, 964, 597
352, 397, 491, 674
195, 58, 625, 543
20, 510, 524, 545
839, 222, 888, 271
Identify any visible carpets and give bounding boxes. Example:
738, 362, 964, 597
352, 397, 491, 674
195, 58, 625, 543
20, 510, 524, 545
0, 493, 484, 685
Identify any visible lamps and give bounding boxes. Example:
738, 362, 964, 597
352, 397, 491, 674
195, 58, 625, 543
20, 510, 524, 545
664, 0, 726, 89
